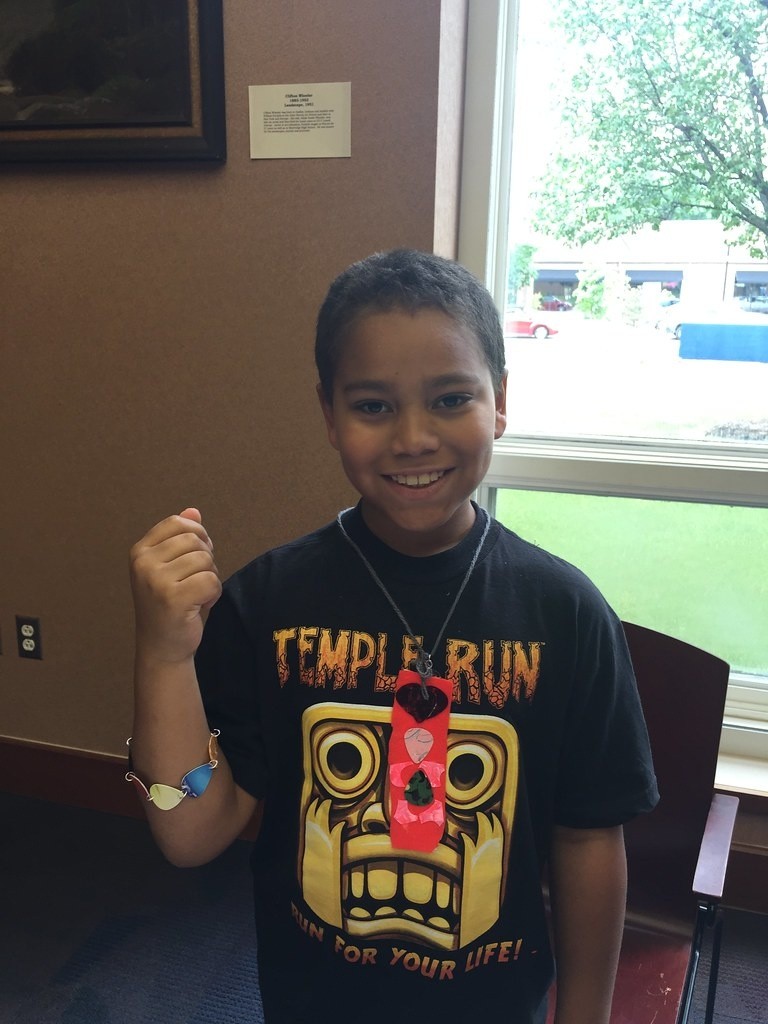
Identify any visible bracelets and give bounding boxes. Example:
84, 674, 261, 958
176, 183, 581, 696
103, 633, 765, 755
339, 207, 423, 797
123, 725, 221, 812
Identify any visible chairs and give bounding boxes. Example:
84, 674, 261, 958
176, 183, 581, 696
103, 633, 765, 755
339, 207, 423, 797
546, 621, 739, 1024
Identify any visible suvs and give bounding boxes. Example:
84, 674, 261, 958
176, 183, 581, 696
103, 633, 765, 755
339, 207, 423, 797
541, 295, 571, 311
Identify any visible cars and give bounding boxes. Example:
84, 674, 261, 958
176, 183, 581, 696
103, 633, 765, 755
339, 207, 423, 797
657, 299, 756, 340
509, 306, 557, 338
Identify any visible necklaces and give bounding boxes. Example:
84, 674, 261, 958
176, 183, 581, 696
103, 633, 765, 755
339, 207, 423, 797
335, 502, 490, 850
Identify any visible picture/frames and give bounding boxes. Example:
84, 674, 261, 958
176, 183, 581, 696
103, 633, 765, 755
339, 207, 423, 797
0, 0, 226, 165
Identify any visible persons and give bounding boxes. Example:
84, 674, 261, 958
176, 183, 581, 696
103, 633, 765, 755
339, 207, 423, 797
124, 245, 661, 1023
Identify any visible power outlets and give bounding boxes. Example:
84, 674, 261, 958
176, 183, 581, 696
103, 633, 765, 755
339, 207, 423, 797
15, 615, 42, 660
